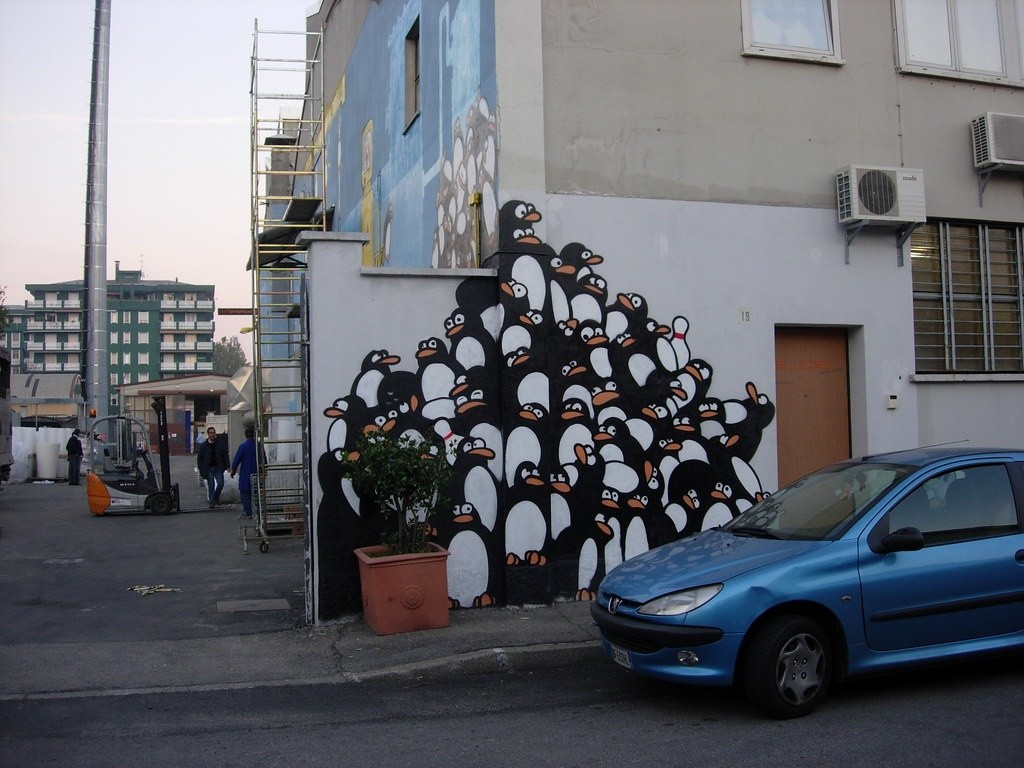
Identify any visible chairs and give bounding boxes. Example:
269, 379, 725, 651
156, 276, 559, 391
104, 457, 131, 473
888, 478, 1024, 535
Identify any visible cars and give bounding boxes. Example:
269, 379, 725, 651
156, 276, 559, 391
588, 447, 1024, 721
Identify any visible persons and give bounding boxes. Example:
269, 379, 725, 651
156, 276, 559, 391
231, 429, 267, 519
197, 427, 231, 508
66, 429, 84, 485
196, 432, 204, 451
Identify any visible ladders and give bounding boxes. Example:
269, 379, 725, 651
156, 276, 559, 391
242, 18, 327, 553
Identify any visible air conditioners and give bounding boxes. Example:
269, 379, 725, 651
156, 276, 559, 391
836, 165, 926, 226
970, 112, 1024, 172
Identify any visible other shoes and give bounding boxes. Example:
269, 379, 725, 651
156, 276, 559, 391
239, 515, 253, 519
208, 504, 215, 509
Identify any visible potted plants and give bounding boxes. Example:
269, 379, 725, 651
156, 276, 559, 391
339, 430, 463, 636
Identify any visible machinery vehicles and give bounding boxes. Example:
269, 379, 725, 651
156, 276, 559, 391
84, 393, 235, 517
